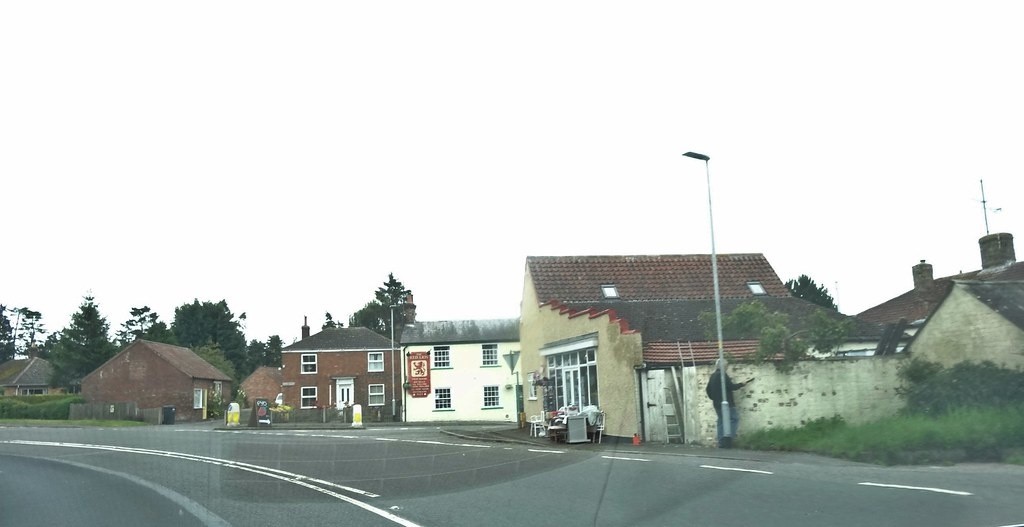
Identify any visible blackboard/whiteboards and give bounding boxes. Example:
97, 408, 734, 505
248, 398, 272, 427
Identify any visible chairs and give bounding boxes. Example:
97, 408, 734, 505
528, 411, 545, 437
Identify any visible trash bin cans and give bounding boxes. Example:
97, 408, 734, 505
162, 405, 176, 424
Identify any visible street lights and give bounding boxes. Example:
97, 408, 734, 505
389, 304, 397, 417
682, 150, 732, 439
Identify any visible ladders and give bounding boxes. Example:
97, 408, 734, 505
660, 387, 683, 445
677, 340, 696, 368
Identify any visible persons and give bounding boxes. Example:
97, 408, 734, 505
706, 358, 746, 449
